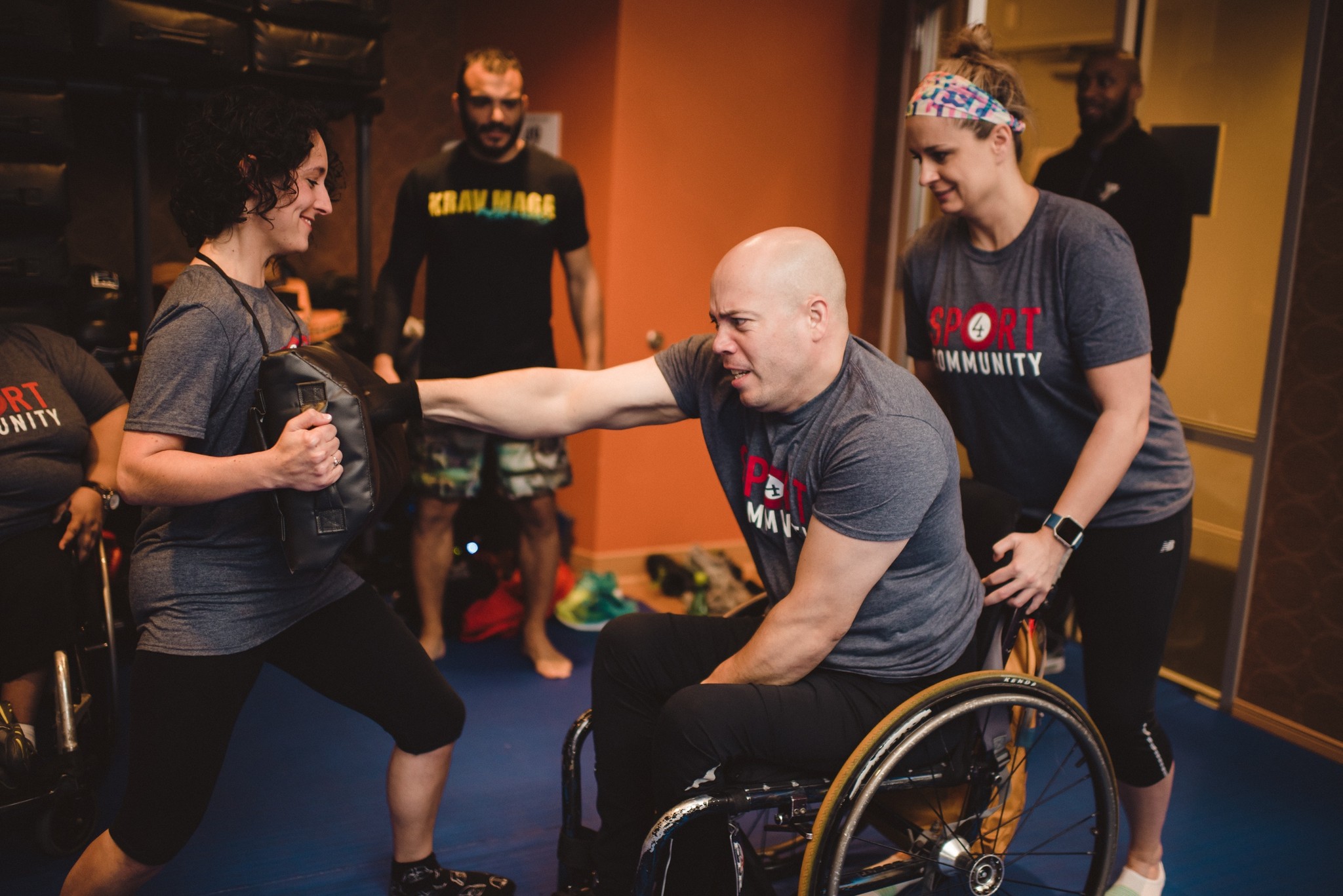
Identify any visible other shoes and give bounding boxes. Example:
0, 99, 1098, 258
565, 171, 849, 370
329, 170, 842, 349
1, 699, 32, 777
555, 570, 638, 631
1107, 859, 1167, 895
391, 854, 516, 896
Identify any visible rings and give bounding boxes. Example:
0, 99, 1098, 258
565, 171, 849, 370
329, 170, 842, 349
331, 453, 339, 467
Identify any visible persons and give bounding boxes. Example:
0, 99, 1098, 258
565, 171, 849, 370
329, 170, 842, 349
0, 39, 598, 896
897, 20, 1193, 896
395, 223, 988, 896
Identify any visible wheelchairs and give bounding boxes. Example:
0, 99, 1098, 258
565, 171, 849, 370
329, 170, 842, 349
556, 476, 1122, 896
0, 510, 132, 867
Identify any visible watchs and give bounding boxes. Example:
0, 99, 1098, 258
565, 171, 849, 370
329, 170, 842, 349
1043, 514, 1083, 553
80, 480, 119, 512
